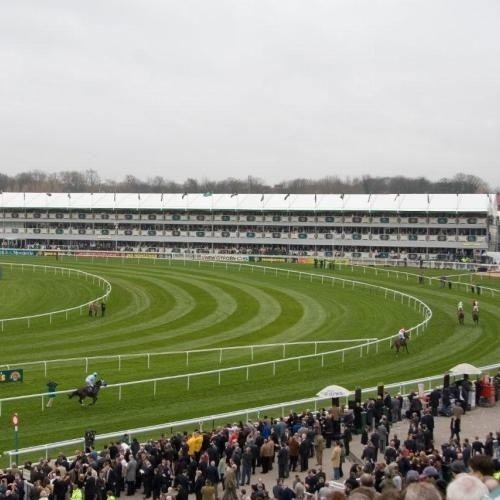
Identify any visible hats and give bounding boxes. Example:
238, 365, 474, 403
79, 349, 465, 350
356, 473, 373, 486
420, 466, 438, 479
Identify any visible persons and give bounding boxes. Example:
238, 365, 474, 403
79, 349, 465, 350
457, 301, 463, 311
399, 328, 407, 341
314, 259, 335, 271
473, 301, 479, 312
89, 301, 106, 316
419, 274, 481, 295
1, 372, 500, 499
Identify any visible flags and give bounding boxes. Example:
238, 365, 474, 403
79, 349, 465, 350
0, 190, 490, 204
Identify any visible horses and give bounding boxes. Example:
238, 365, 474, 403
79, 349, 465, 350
458, 309, 464, 325
472, 301, 479, 327
391, 330, 410, 354
68, 377, 107, 406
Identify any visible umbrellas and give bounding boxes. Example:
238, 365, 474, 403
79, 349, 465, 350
446, 363, 482, 376
